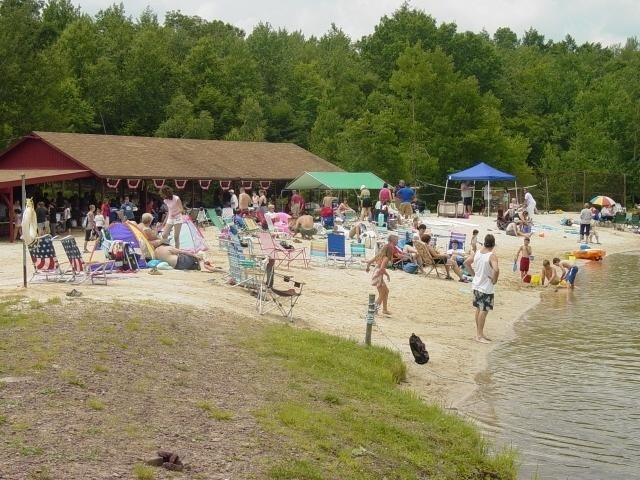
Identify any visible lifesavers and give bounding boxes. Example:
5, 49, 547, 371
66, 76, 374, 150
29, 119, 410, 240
273, 212, 292, 233
574, 249, 605, 260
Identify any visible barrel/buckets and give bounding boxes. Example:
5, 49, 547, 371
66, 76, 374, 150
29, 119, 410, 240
531, 274, 540, 285
568, 254, 575, 262
632, 214, 640, 222
615, 211, 627, 223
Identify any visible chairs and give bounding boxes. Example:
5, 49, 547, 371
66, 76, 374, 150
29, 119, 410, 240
191, 208, 466, 323
592, 210, 640, 230
102, 229, 140, 271
89, 222, 103, 262
61, 235, 115, 285
497, 209, 504, 228
27, 234, 71, 282
